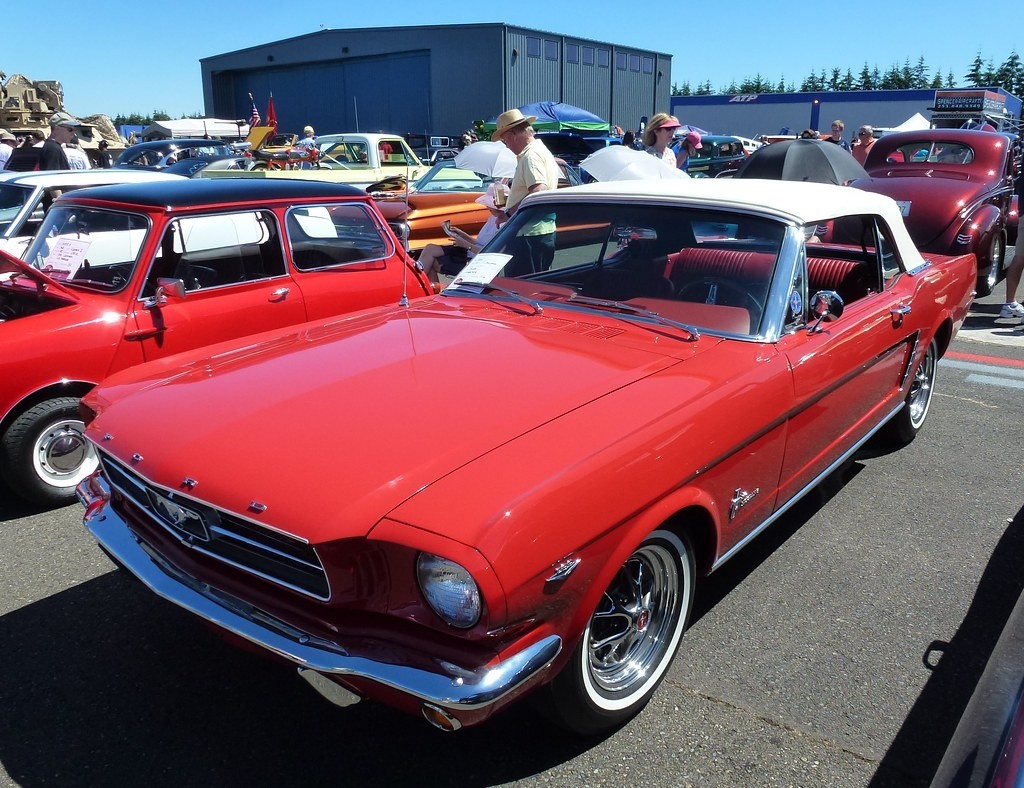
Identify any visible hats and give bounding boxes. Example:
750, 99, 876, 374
491, 109, 536, 143
304, 126, 314, 133
2, 133, 19, 145
97, 140, 109, 147
687, 131, 703, 148
476, 183, 510, 211
653, 120, 682, 127
48, 112, 81, 126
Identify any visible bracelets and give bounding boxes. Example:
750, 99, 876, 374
468, 243, 473, 251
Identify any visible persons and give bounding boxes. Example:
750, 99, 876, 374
199, 134, 218, 156
380, 142, 396, 166
758, 136, 770, 146
296, 126, 318, 145
798, 128, 816, 140
822, 120, 852, 152
0, 112, 114, 170
622, 130, 639, 151
1000, 152, 1024, 324
635, 112, 681, 170
488, 106, 561, 277
851, 125, 878, 165
459, 130, 479, 151
675, 132, 704, 175
613, 125, 622, 134
415, 178, 511, 293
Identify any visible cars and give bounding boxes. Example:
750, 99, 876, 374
732, 135, 763, 154
0, 132, 615, 284
73, 177, 974, 741
850, 126, 902, 152
669, 137, 750, 179
0, 173, 444, 511
847, 124, 1024, 297
762, 133, 834, 145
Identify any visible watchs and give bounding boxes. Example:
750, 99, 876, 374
501, 206, 513, 221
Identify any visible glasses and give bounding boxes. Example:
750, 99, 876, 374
803, 136, 813, 139
500, 134, 515, 145
657, 127, 675, 131
59, 125, 76, 132
859, 132, 868, 136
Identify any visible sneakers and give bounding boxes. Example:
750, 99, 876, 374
1021, 316, 1024, 323
999, 301, 1024, 318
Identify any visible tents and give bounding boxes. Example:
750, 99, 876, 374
483, 101, 610, 137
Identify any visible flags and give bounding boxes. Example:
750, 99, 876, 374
250, 100, 262, 128
264, 97, 281, 141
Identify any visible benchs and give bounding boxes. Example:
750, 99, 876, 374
669, 248, 868, 322
263, 242, 373, 276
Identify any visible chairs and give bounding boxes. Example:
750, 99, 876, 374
156, 262, 217, 291
334, 155, 349, 163
682, 275, 768, 336
582, 267, 677, 302
